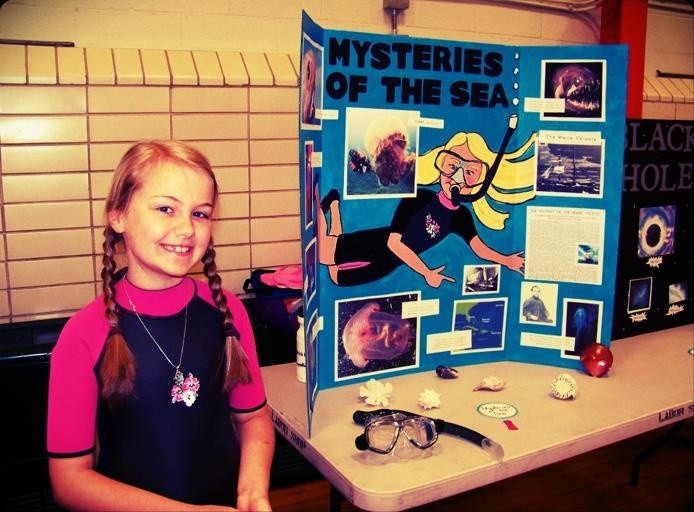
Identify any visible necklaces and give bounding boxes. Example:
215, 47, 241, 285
121, 271, 201, 409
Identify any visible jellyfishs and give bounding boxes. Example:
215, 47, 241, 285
342, 300, 410, 368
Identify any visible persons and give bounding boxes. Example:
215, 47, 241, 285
44, 141, 277, 512
314, 132, 539, 287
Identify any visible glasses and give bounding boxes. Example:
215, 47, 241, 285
355, 413, 441, 454
434, 149, 491, 189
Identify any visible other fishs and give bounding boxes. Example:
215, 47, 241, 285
554, 65, 600, 114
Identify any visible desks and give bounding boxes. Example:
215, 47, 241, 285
257, 321, 693, 511
